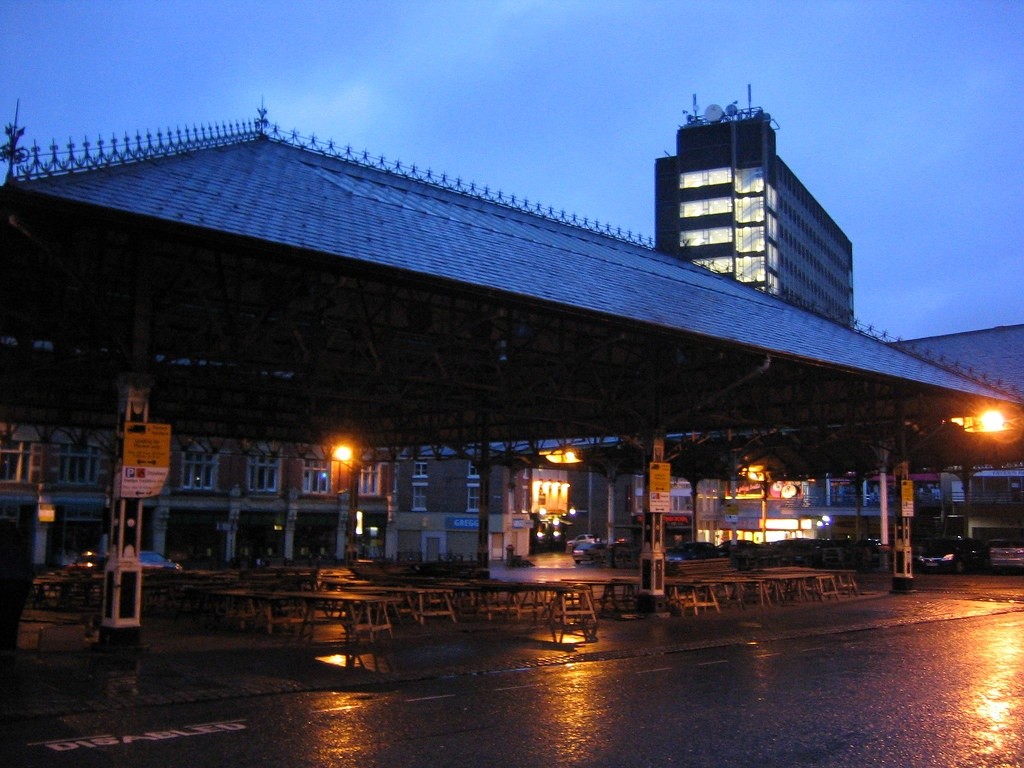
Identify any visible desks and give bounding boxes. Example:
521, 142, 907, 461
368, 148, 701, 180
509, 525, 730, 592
30, 569, 859, 643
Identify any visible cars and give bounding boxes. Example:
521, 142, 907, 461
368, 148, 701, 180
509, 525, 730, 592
712, 538, 882, 568
567, 534, 601, 547
911, 536, 934, 560
982, 539, 1024, 575
572, 543, 606, 564
665, 541, 724, 566
139, 551, 183, 571
917, 536, 987, 575
